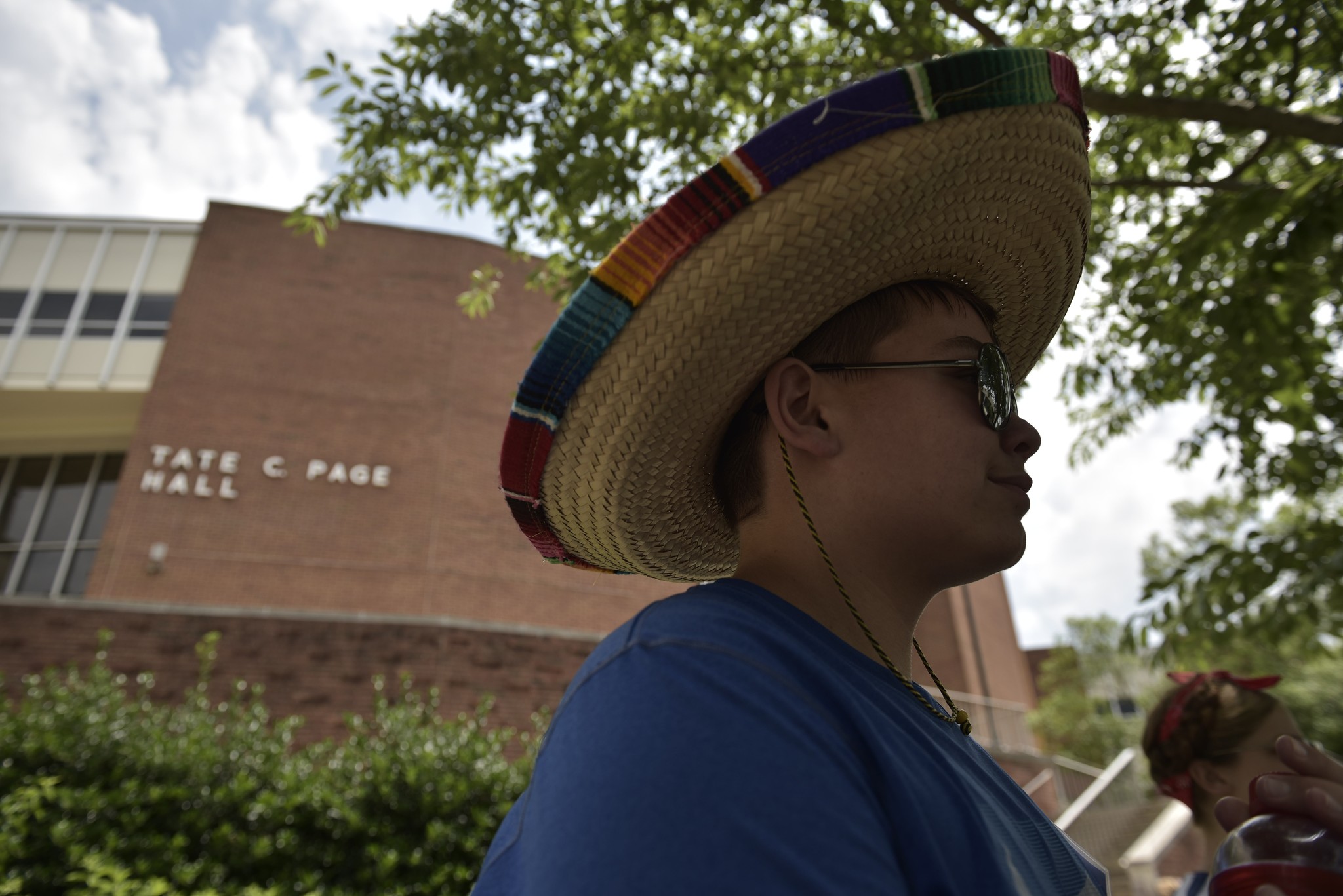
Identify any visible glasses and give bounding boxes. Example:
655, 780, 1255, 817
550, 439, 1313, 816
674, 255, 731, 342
758, 341, 1018, 430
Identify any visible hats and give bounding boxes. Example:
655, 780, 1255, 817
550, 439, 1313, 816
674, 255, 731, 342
499, 49, 1106, 580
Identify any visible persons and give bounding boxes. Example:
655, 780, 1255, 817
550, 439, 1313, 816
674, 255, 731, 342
1142, 671, 1306, 896
474, 47, 1343, 896
